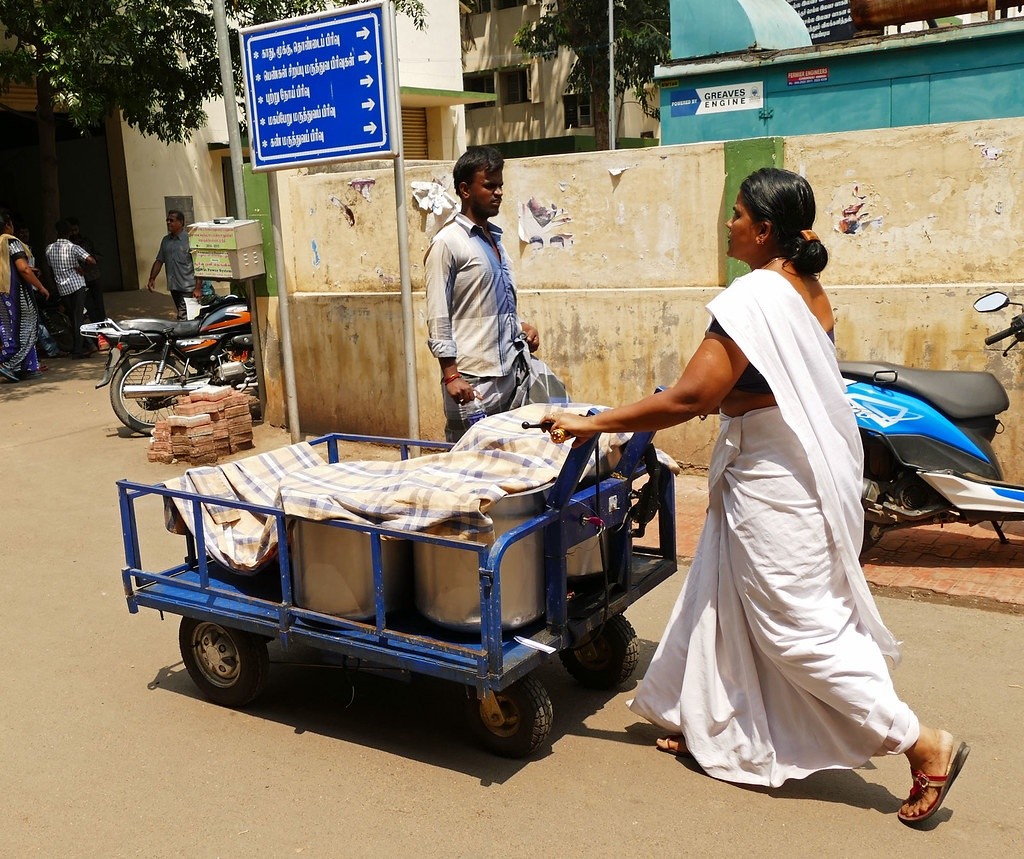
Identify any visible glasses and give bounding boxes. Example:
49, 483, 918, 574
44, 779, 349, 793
166, 218, 178, 222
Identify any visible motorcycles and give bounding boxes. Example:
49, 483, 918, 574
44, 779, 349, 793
79, 281, 257, 436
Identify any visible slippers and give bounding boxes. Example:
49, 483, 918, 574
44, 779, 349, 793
656, 732, 694, 757
898, 740, 971, 822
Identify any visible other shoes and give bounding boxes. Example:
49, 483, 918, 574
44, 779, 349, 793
22, 372, 43, 379
0, 364, 20, 381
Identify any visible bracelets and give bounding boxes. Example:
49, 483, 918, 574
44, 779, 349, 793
149, 277, 155, 281
444, 372, 461, 385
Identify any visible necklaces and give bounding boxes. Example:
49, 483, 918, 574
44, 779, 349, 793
762, 257, 788, 269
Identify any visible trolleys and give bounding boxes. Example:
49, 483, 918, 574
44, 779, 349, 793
113, 381, 680, 757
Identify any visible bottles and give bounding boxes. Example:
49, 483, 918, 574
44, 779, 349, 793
466, 391, 488, 428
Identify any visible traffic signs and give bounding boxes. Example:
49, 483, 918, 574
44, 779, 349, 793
239, 0, 400, 167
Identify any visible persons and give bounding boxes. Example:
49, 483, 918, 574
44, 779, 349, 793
148, 210, 202, 321
423, 144, 540, 444
0, 214, 49, 382
45, 221, 96, 359
544, 167, 970, 826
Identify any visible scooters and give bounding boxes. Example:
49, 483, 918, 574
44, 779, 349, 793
829, 287, 1024, 559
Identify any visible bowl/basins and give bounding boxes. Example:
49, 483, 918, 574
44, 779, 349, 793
487, 477, 558, 515
586, 442, 624, 477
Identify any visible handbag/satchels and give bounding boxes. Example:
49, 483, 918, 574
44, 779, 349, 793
183, 296, 210, 321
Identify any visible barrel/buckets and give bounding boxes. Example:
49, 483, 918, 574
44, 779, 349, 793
288, 517, 546, 630
566, 533, 603, 577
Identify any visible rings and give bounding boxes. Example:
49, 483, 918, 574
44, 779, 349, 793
551, 414, 555, 418
459, 399, 464, 403
